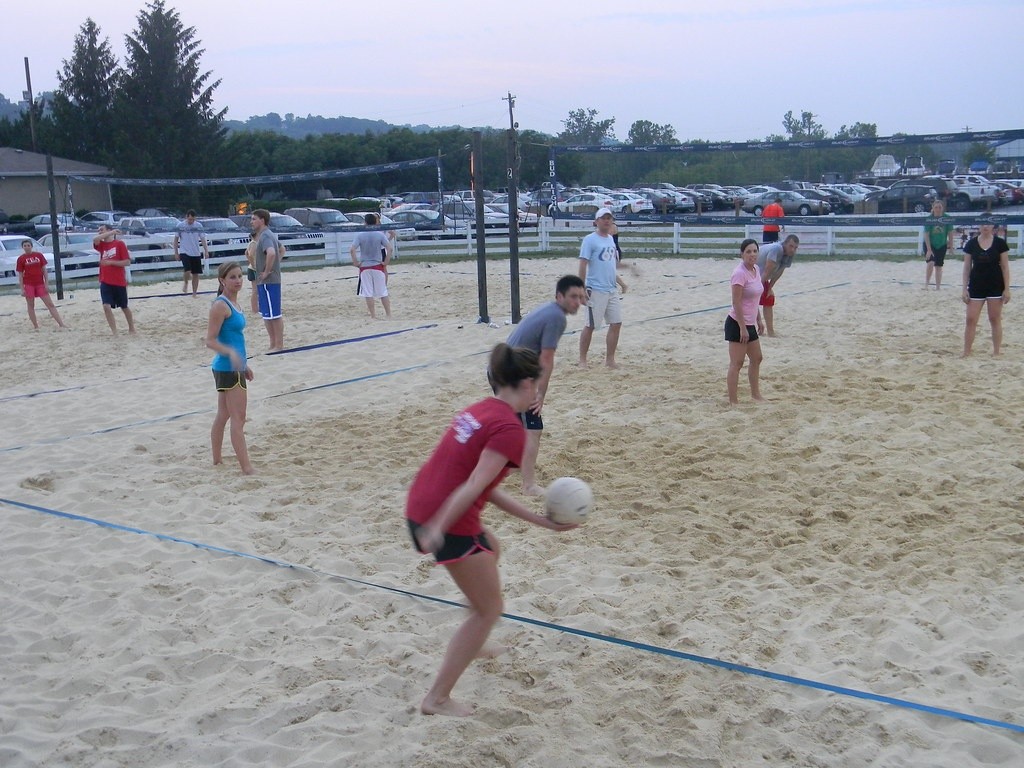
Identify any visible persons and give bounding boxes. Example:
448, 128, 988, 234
406, 344, 579, 718
93, 224, 136, 337
923, 200, 954, 290
959, 213, 1010, 357
374, 212, 396, 285
174, 210, 209, 297
351, 214, 393, 320
756, 235, 799, 337
206, 262, 258, 474
487, 274, 585, 498
725, 239, 769, 405
245, 231, 262, 316
16, 239, 68, 329
251, 211, 286, 350
609, 217, 622, 263
762, 199, 785, 244
579, 208, 628, 369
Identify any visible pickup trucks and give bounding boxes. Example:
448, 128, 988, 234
940, 178, 997, 211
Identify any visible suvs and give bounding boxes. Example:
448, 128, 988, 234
887, 178, 959, 209
953, 175, 989, 185
795, 181, 886, 213
283, 207, 366, 231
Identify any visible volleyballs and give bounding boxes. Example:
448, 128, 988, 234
545, 477, 594, 526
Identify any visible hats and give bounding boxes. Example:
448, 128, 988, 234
595, 208, 613, 220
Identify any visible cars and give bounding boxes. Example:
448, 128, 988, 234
743, 186, 831, 216
325, 189, 541, 240
0, 192, 325, 282
865, 185, 937, 213
528, 182, 751, 217
953, 179, 1024, 206
